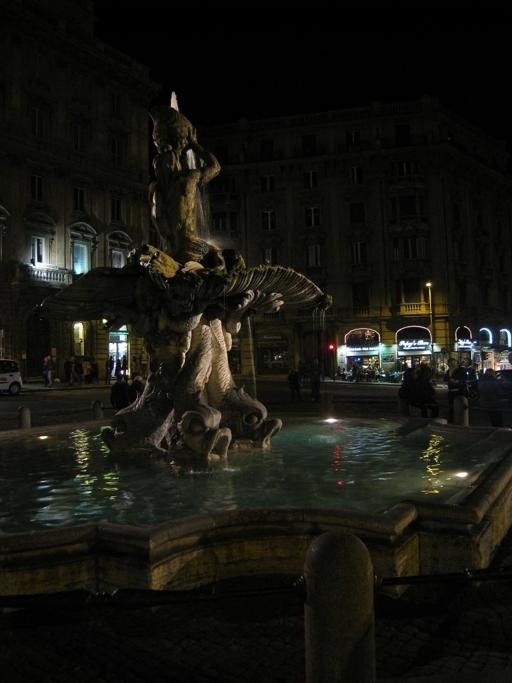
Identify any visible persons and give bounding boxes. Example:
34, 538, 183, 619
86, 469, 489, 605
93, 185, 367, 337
111, 375, 129, 411
39, 351, 139, 384
129, 376, 145, 403
145, 127, 229, 266
287, 354, 506, 427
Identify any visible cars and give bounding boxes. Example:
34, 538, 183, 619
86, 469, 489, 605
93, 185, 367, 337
0, 359, 23, 396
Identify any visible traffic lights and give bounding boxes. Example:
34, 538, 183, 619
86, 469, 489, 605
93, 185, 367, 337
328, 339, 334, 353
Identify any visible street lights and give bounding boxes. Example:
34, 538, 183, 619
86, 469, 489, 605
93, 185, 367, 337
425, 281, 435, 382
473, 337, 483, 376
